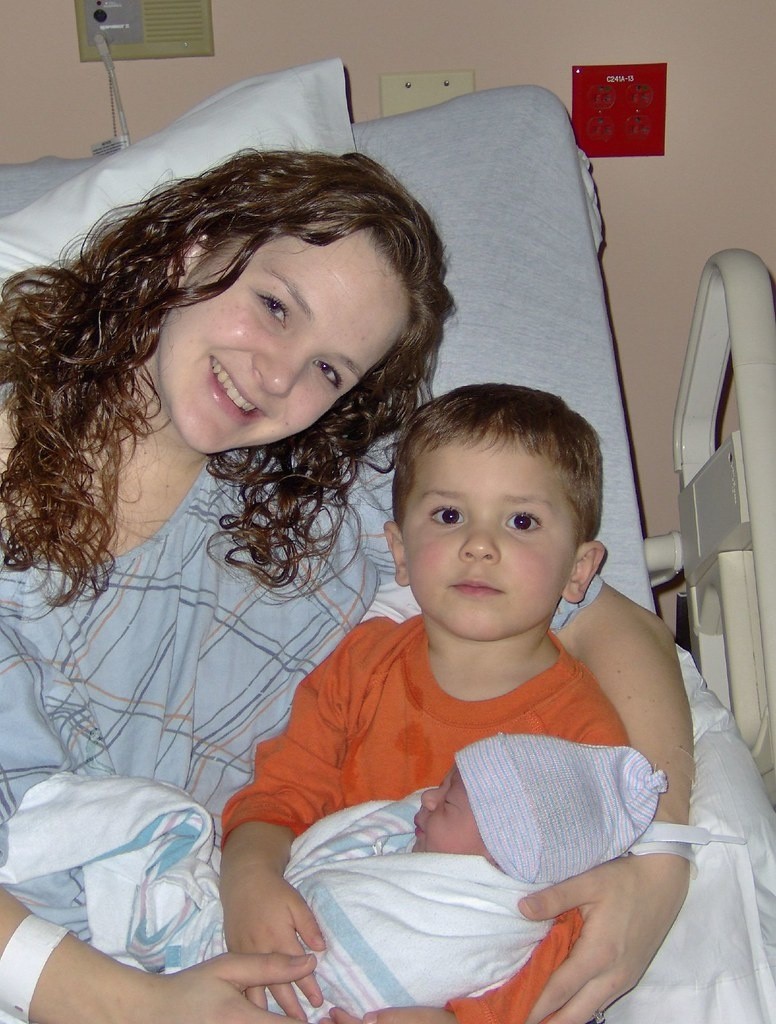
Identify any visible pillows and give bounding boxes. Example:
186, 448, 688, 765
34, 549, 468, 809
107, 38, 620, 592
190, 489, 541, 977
2, 61, 351, 292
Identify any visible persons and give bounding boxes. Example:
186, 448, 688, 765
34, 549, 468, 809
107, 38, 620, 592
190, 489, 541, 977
0, 151, 695, 1024
228, 382, 628, 1024
166, 731, 611, 1024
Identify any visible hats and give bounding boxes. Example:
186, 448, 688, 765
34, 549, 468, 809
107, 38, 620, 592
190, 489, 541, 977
453, 730, 668, 882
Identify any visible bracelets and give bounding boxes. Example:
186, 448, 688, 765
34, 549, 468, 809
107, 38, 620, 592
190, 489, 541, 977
622, 823, 747, 880
0, 913, 70, 1024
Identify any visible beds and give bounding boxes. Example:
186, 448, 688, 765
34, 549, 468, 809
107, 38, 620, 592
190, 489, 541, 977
2, 61, 776, 1024
